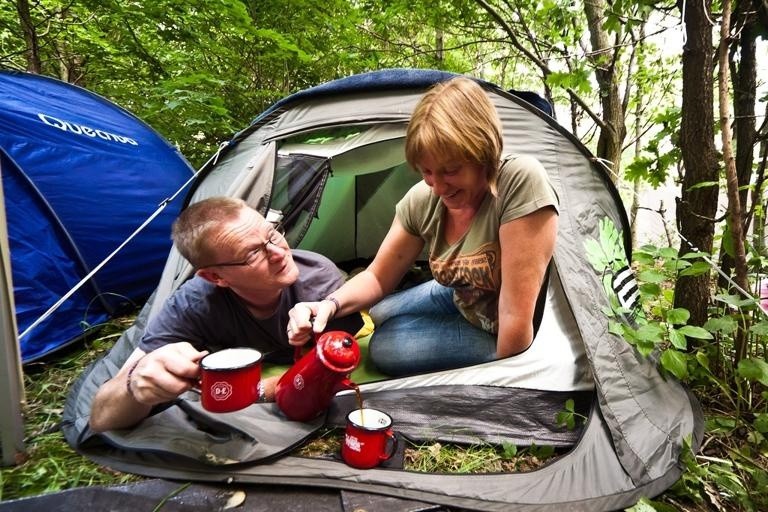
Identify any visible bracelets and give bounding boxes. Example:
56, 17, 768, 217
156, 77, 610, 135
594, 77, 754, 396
323, 297, 340, 318
127, 352, 148, 395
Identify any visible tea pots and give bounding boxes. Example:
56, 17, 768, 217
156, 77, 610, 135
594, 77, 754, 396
273, 330, 362, 421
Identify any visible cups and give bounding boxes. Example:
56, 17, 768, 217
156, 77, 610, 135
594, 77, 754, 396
191, 346, 265, 414
341, 407, 397, 468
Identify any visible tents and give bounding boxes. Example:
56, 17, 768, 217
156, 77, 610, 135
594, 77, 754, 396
1, 67, 198, 366
62, 67, 705, 512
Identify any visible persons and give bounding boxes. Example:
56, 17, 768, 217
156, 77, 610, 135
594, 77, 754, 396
89, 198, 377, 434
287, 79, 561, 375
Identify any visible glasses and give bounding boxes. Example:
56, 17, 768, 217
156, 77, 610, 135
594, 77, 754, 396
199, 220, 287, 268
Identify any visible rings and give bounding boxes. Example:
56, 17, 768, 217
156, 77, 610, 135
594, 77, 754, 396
286, 327, 291, 334
